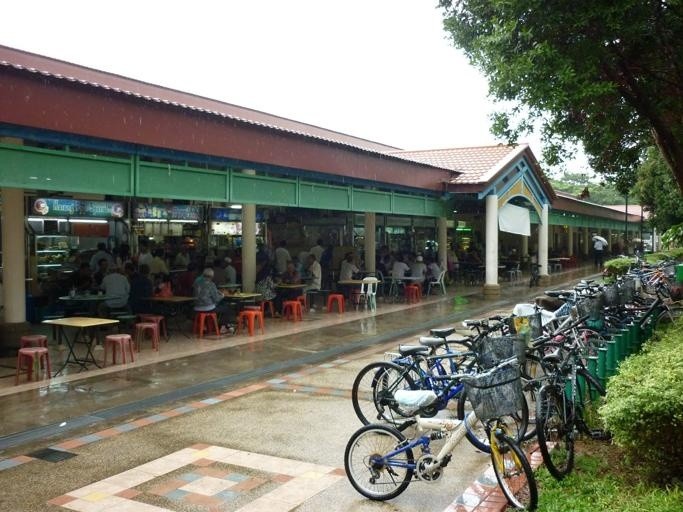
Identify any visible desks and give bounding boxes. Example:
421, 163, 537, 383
382, 276, 422, 304
59, 294, 123, 313
223, 293, 263, 335
338, 278, 384, 309
141, 296, 195, 317
37, 318, 122, 378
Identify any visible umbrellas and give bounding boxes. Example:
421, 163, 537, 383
592, 235, 608, 246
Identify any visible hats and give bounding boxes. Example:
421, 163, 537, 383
416, 256, 424, 261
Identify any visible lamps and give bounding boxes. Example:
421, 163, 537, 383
27, 216, 67, 222
70, 218, 109, 224
136, 217, 165, 223
173, 219, 199, 224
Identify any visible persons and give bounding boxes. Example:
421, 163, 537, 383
56, 249, 81, 294
98, 263, 131, 330
300, 255, 320, 310
411, 256, 427, 292
310, 239, 326, 263
192, 268, 231, 335
94, 260, 106, 285
340, 252, 354, 267
276, 241, 290, 273
319, 244, 334, 285
152, 280, 175, 317
392, 258, 409, 293
424, 260, 440, 294
116, 244, 131, 270
70, 263, 90, 290
137, 241, 151, 268
232, 248, 241, 283
338, 257, 359, 296
124, 264, 138, 281
375, 256, 384, 276
90, 242, 112, 267
278, 263, 299, 283
179, 263, 197, 294
384, 256, 393, 272
132, 264, 151, 309
257, 268, 282, 317
224, 257, 236, 292
594, 239, 603, 268
360, 252, 364, 266
213, 260, 225, 283
380, 248, 396, 265
175, 247, 192, 267
151, 252, 168, 283
257, 244, 276, 267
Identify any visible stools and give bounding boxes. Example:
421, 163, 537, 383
554, 264, 562, 273
13, 347, 51, 385
504, 271, 516, 282
21, 334, 48, 370
327, 294, 345, 313
403, 286, 420, 304
305, 291, 318, 312
136, 322, 159, 354
192, 310, 220, 340
514, 270, 522, 282
548, 265, 551, 274
317, 289, 332, 310
282, 300, 303, 324
242, 305, 261, 328
142, 315, 170, 344
102, 333, 135, 368
237, 309, 264, 338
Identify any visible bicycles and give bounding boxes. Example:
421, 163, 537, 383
351, 317, 529, 455
427, 255, 682, 402
371, 320, 510, 425
530, 313, 615, 481
344, 348, 538, 511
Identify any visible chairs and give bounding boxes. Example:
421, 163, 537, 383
294, 295, 307, 311
351, 276, 378, 314
387, 269, 406, 296
257, 300, 276, 318
426, 270, 446, 296
408, 282, 422, 299
